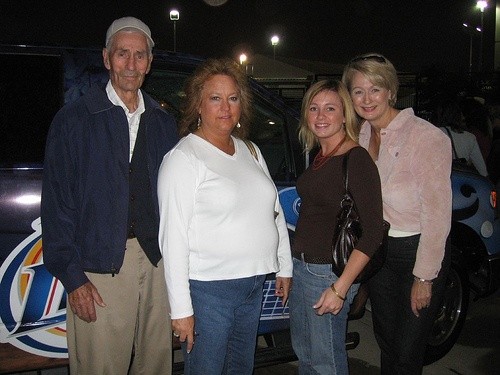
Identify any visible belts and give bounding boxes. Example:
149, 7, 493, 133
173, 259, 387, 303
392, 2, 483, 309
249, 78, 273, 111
127, 229, 136, 238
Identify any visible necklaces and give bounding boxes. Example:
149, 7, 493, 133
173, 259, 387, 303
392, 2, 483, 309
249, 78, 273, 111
310, 134, 346, 170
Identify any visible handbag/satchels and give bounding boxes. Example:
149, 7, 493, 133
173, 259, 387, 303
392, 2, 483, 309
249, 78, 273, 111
452, 157, 480, 174
332, 146, 390, 284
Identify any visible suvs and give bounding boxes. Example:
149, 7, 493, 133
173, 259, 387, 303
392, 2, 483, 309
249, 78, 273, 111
0, 42, 493, 375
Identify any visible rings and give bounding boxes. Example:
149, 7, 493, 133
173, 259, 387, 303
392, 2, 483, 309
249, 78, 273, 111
280, 287, 283, 289
173, 333, 179, 337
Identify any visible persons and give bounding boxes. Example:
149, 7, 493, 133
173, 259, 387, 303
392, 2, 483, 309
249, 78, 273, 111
39, 16, 180, 375
340, 53, 453, 374
156, 58, 293, 375
286, 78, 384, 374
433, 97, 500, 194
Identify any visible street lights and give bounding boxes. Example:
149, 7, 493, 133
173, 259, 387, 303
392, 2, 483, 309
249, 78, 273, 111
170, 9, 180, 52
476, 0, 489, 62
463, 23, 474, 73
269, 34, 280, 59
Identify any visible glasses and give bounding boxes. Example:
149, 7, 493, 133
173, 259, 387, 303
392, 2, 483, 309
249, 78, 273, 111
352, 56, 386, 63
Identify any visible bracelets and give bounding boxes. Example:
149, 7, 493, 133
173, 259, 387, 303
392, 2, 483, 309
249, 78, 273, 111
330, 283, 345, 301
414, 276, 433, 285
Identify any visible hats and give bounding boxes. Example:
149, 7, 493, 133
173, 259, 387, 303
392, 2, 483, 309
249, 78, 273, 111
105, 17, 154, 47
